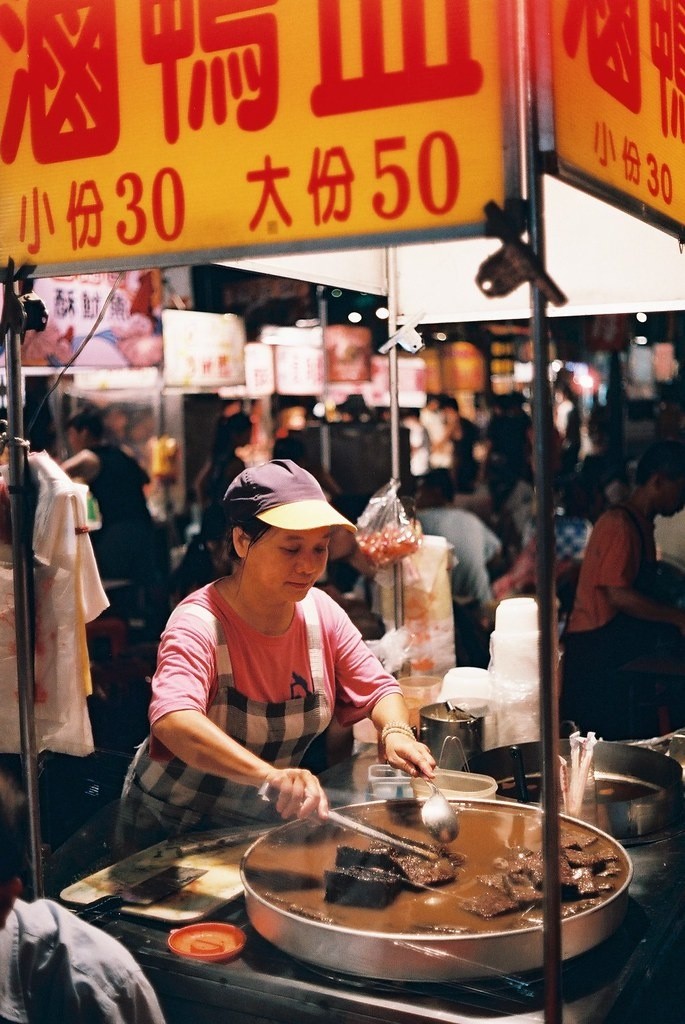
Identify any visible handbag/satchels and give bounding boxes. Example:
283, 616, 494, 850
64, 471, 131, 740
355, 480, 420, 570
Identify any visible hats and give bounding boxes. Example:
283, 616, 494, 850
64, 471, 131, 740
223, 459, 357, 535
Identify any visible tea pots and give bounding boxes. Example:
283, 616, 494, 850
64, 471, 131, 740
411, 704, 485, 771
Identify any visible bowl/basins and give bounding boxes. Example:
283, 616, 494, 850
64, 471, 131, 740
366, 764, 414, 799
430, 770, 499, 800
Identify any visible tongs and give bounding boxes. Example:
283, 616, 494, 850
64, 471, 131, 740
259, 783, 439, 863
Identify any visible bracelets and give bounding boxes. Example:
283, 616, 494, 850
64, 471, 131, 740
381, 721, 417, 742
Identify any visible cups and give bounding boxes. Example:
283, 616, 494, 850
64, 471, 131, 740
399, 676, 442, 727
441, 596, 537, 753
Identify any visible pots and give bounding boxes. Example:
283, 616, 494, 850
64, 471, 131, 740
240, 798, 634, 980
463, 739, 682, 845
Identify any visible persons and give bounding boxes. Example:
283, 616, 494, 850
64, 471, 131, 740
118, 458, 438, 856
0, 774, 167, 1024
20, 295, 191, 366
563, 437, 685, 737
59, 369, 685, 669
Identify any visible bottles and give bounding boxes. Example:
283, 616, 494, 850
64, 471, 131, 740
353, 720, 378, 744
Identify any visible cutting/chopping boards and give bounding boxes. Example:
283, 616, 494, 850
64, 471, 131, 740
59, 823, 274, 924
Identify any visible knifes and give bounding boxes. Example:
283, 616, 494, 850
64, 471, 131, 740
75, 865, 207, 918
